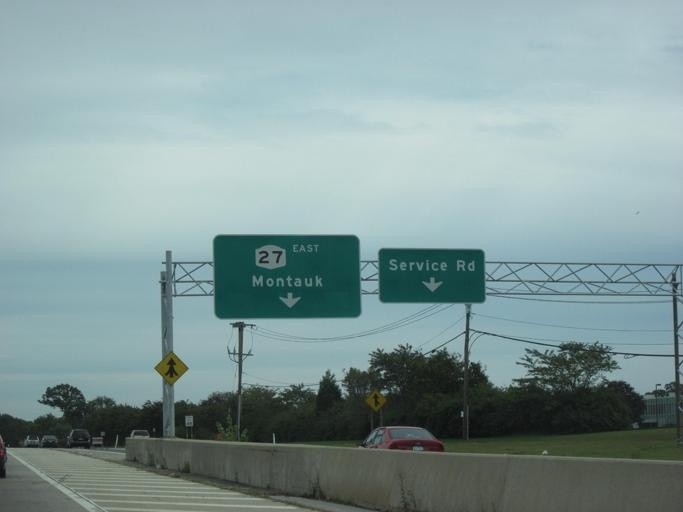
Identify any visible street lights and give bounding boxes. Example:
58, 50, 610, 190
655, 383, 661, 428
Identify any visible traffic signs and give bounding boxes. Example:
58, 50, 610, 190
379, 250, 485, 303
213, 235, 361, 318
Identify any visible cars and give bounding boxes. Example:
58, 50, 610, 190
24, 434, 57, 447
131, 430, 150, 438
359, 426, 444, 451
0, 435, 7, 477
65, 429, 91, 449
